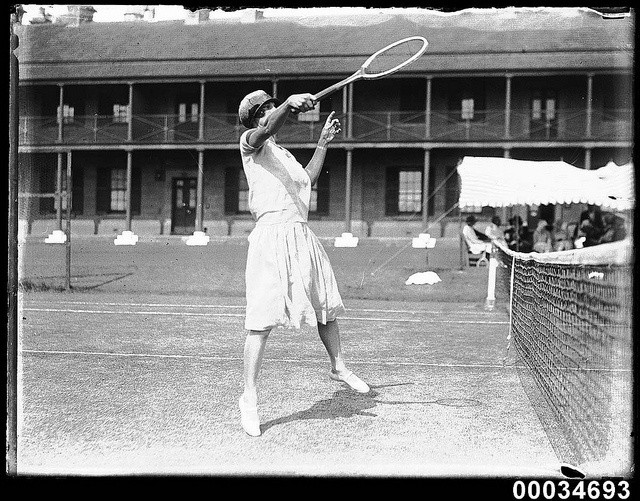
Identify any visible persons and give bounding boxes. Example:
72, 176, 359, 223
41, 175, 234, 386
464, 204, 618, 254
239, 90, 371, 436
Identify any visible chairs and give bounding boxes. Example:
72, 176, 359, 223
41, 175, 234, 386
461, 234, 487, 267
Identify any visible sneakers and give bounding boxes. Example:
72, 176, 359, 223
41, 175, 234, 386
327, 360, 370, 395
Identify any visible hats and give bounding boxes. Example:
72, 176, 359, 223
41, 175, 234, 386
509, 216, 522, 226
465, 216, 478, 222
239, 90, 283, 129
